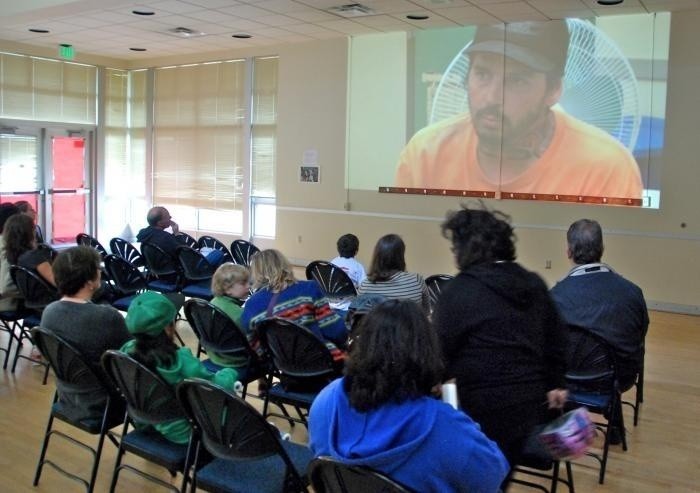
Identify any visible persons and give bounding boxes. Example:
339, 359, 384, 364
333, 234, 431, 314
430, 208, 572, 492
307, 299, 511, 493
137, 207, 226, 280
117, 292, 238, 443
39, 245, 135, 425
1, 200, 126, 363
202, 247, 353, 383
393, 20, 642, 200
549, 219, 651, 395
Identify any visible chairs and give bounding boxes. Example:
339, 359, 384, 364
507, 403, 576, 492
251, 316, 342, 429
306, 454, 415, 491
29, 324, 124, 492
178, 374, 315, 491
620, 357, 646, 426
560, 321, 627, 483
98, 348, 197, 492
182, 297, 296, 432
1, 224, 456, 387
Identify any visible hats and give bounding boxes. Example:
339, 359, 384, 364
125, 289, 183, 335
463, 17, 569, 70
